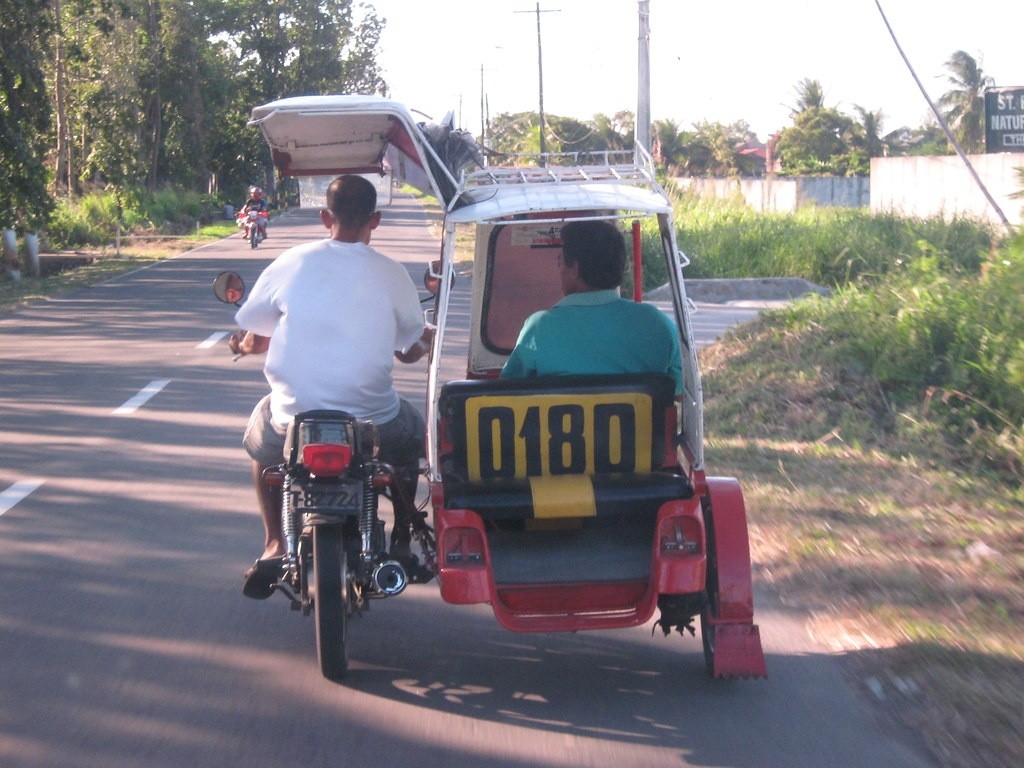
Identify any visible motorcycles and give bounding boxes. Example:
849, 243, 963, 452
213, 96, 769, 682
240, 204, 266, 251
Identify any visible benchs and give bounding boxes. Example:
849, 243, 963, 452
435, 373, 672, 493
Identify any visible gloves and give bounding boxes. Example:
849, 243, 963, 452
239, 214, 246, 218
262, 212, 267, 217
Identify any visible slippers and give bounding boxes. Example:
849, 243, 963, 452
242, 555, 285, 600
388, 552, 419, 570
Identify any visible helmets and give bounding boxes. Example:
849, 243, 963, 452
250, 187, 261, 193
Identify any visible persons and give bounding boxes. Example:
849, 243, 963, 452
225, 175, 438, 600
235, 185, 272, 239
224, 275, 245, 301
495, 222, 683, 402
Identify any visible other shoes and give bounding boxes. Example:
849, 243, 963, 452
262, 232, 266, 239
241, 234, 247, 239
